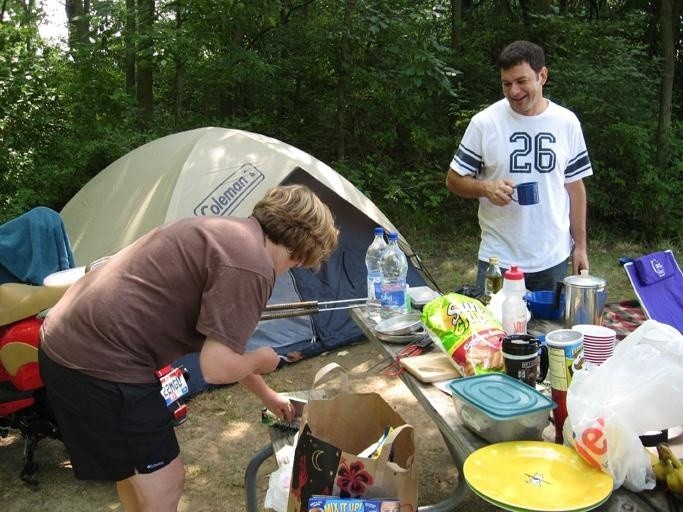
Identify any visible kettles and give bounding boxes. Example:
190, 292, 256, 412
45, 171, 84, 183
553, 269, 607, 326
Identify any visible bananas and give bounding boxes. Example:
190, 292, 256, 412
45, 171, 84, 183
645, 443, 683, 495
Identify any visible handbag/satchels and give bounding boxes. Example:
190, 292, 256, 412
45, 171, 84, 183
286, 361, 418, 512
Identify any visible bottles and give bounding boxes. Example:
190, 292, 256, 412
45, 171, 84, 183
364, 226, 386, 311
484, 256, 503, 305
544, 329, 583, 438
502, 265, 528, 335
378, 232, 408, 322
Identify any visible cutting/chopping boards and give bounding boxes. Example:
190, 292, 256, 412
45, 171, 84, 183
398, 352, 462, 384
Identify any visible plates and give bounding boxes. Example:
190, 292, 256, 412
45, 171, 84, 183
462, 439, 614, 511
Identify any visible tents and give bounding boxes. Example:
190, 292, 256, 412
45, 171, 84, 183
56, 125, 445, 402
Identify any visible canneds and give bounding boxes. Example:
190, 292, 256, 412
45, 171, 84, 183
546, 327, 586, 391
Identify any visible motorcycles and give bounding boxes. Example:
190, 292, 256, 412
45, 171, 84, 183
1, 208, 191, 492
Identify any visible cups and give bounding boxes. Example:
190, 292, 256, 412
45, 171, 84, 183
571, 324, 617, 367
508, 181, 540, 206
501, 333, 550, 391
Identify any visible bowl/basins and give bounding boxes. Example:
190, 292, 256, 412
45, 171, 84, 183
523, 290, 565, 320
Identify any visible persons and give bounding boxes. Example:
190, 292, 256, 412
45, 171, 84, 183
445, 39, 595, 295
37, 183, 342, 512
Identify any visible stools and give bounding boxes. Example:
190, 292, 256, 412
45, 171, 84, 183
245, 390, 328, 512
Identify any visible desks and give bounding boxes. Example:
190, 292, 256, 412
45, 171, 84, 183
348, 304, 683, 512
